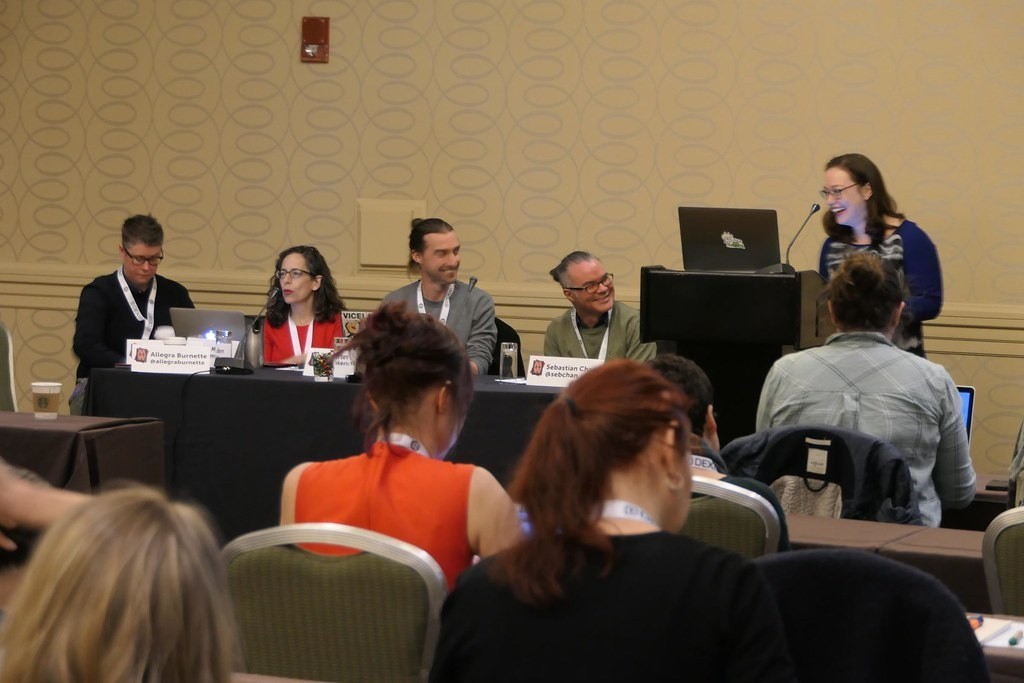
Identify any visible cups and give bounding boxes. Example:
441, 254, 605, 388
499, 342, 517, 384
32, 382, 63, 421
312, 352, 334, 382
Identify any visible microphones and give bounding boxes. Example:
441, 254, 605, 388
214, 288, 280, 375
781, 204, 821, 274
452, 276, 478, 335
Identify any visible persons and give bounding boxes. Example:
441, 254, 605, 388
280, 298, 526, 594
68, 215, 196, 416
262, 245, 344, 365
0, 488, 241, 683
818, 153, 944, 359
378, 217, 498, 375
543, 250, 657, 364
755, 252, 977, 527
427, 357, 799, 683
644, 352, 789, 561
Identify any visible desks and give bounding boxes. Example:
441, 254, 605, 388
0, 365, 1024, 683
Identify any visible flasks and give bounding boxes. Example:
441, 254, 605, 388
245, 317, 264, 368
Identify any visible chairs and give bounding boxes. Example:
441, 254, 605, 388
220, 522, 448, 683
982, 506, 1024, 616
678, 475, 781, 560
749, 547, 989, 683
719, 426, 924, 527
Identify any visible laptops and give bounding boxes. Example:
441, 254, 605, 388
170, 308, 246, 359
678, 207, 782, 273
957, 384, 976, 450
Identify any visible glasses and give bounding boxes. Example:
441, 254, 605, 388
819, 181, 861, 201
275, 268, 315, 279
123, 245, 167, 266
567, 273, 614, 294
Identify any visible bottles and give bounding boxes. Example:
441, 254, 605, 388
216, 329, 232, 359
